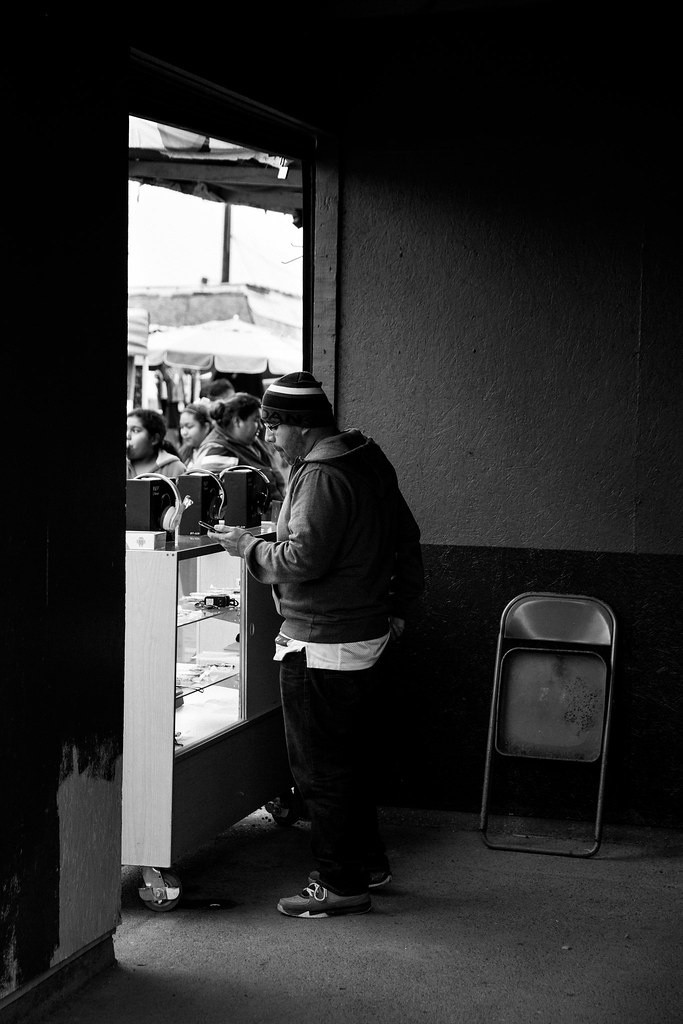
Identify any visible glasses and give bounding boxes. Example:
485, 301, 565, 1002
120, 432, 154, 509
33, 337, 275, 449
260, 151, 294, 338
264, 421, 282, 433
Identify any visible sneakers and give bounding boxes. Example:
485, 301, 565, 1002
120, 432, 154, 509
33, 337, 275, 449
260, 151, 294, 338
309, 869, 392, 889
277, 885, 373, 918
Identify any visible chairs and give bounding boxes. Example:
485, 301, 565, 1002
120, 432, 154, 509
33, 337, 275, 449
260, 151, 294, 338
477, 591, 617, 858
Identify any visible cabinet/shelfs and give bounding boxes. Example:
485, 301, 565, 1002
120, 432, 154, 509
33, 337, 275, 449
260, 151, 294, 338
121, 525, 293, 912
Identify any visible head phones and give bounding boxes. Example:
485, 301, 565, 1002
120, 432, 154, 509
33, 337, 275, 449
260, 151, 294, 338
133, 472, 184, 531
177, 468, 229, 520
219, 465, 274, 515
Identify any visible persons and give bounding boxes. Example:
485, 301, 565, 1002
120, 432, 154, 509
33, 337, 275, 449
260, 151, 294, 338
173, 397, 214, 472
206, 369, 425, 918
126, 409, 186, 478
199, 392, 286, 520
198, 378, 235, 398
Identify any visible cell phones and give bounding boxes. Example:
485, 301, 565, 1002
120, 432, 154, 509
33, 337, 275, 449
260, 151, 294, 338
198, 521, 223, 534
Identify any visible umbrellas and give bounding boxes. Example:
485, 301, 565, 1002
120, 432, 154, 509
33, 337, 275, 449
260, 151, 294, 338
150, 312, 303, 374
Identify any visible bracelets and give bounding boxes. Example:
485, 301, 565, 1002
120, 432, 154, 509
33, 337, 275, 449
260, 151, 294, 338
237, 531, 253, 558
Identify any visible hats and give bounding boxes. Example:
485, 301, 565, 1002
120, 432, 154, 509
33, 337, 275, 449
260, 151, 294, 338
260, 371, 335, 429
